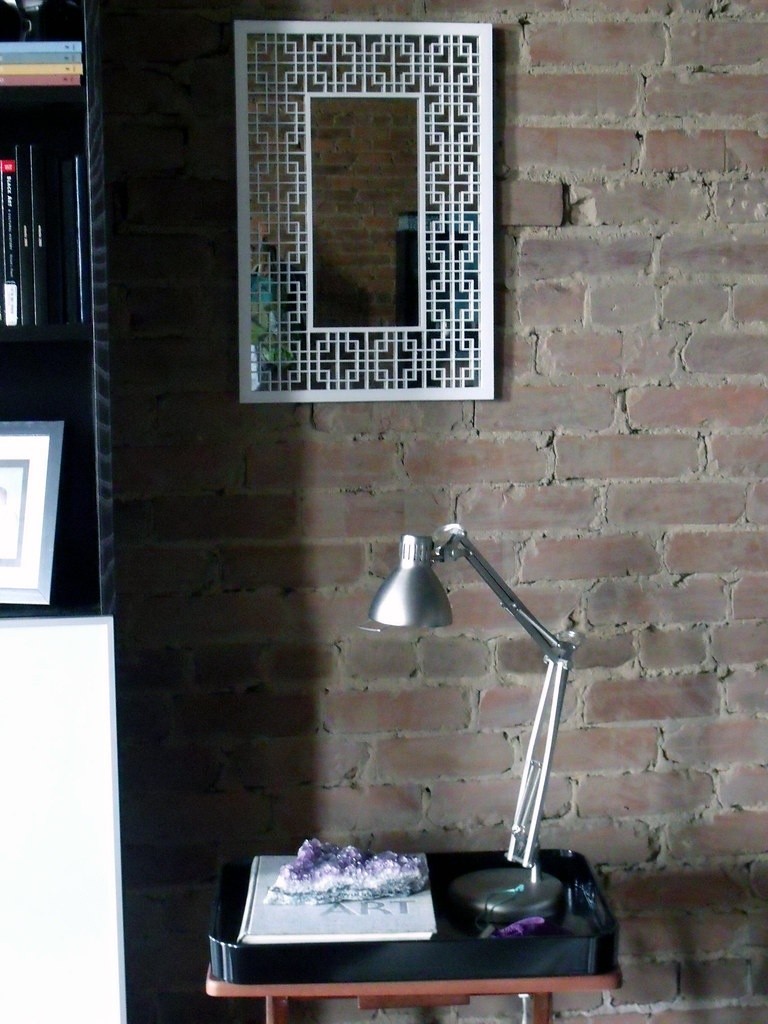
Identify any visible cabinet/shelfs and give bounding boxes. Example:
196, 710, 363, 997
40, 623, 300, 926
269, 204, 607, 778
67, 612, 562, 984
0, 0, 127, 1024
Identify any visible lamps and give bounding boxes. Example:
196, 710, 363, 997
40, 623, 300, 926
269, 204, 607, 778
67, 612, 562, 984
358, 524, 585, 924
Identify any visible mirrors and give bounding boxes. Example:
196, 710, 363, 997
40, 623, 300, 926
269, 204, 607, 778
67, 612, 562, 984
233, 20, 494, 403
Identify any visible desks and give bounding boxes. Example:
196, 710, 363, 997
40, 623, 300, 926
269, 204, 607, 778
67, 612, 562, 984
204, 849, 623, 1024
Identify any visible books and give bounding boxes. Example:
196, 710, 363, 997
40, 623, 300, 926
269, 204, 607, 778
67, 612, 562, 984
0, 144, 90, 327
0, 41, 84, 86
237, 856, 438, 944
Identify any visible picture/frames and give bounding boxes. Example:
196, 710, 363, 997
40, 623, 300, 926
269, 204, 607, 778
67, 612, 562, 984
0, 421, 64, 604
